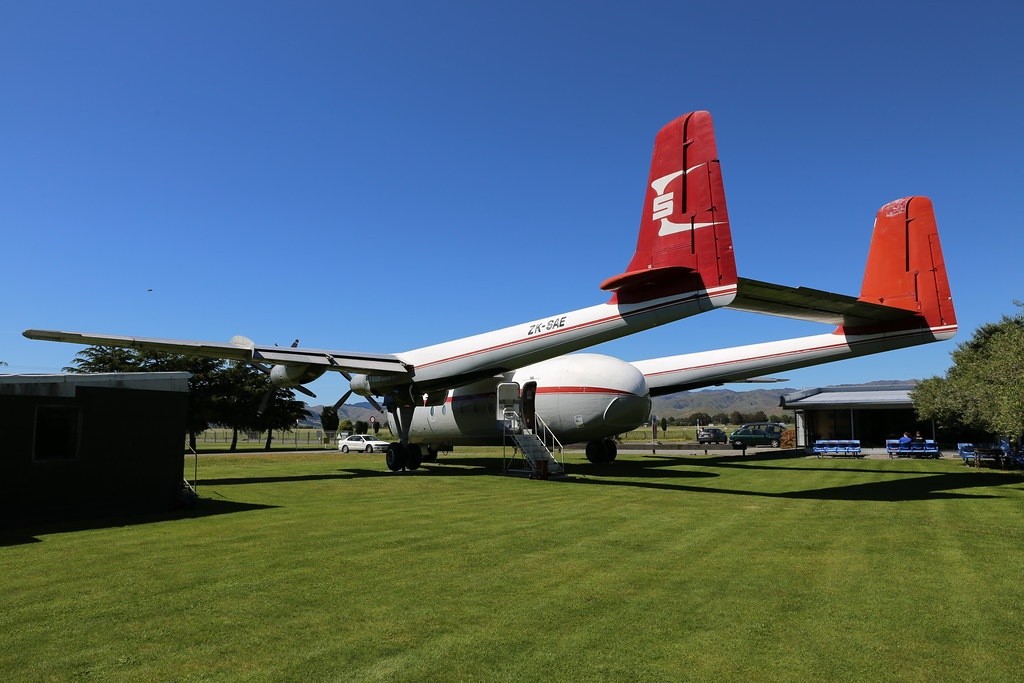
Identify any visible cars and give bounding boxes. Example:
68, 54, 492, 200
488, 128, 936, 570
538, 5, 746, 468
338, 434, 391, 453
696, 429, 728, 445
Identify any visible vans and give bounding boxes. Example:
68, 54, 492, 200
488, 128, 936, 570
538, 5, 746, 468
740, 422, 787, 432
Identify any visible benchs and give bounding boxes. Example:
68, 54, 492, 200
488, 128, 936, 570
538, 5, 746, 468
813, 439, 861, 457
886, 439, 938, 458
958, 442, 1024, 468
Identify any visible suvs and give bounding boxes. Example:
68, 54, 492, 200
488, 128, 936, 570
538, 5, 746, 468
729, 429, 781, 448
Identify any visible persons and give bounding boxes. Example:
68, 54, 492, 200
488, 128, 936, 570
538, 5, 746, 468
896, 432, 913, 458
911, 431, 925, 458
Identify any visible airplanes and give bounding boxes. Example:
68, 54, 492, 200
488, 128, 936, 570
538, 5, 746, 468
21, 110, 957, 476
